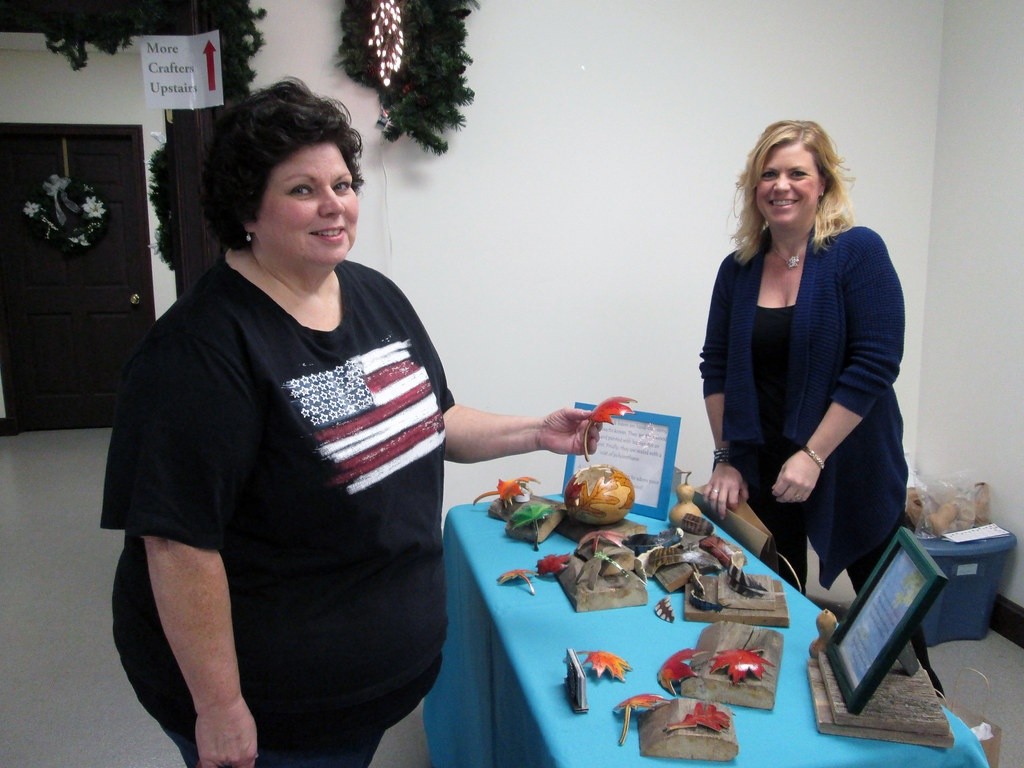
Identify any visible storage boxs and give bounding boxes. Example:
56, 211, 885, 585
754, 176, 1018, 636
920, 522, 1017, 647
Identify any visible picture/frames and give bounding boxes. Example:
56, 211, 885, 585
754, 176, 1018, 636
561, 402, 682, 519
827, 526, 949, 715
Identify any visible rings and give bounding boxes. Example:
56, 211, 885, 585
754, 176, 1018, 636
795, 494, 801, 501
712, 488, 719, 492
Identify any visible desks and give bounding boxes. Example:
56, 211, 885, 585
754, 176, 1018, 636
426, 492, 990, 768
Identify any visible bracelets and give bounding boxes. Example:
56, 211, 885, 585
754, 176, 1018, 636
712, 448, 731, 472
802, 445, 825, 469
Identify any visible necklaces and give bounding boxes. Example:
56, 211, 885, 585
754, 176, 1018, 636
772, 247, 800, 269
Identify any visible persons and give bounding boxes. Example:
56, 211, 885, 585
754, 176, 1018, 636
100, 77, 602, 767
700, 120, 945, 699
